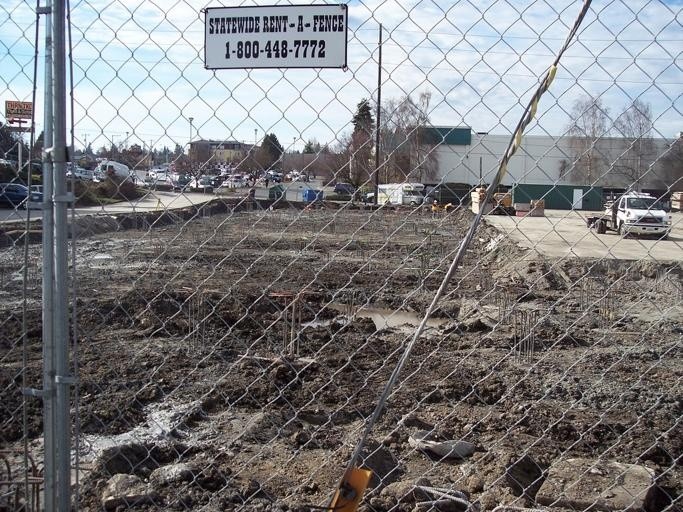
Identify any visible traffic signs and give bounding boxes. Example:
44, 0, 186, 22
5, 101, 34, 119
4, 127, 35, 134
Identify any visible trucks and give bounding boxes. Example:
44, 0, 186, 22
376, 183, 426, 208
585, 193, 672, 240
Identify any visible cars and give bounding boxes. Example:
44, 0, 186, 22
66, 159, 137, 185
0, 184, 44, 211
142, 156, 308, 193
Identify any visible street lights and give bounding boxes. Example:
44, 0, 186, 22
254, 128, 258, 156
189, 117, 194, 152
292, 136, 296, 156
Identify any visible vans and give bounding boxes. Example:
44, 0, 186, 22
332, 183, 357, 194
